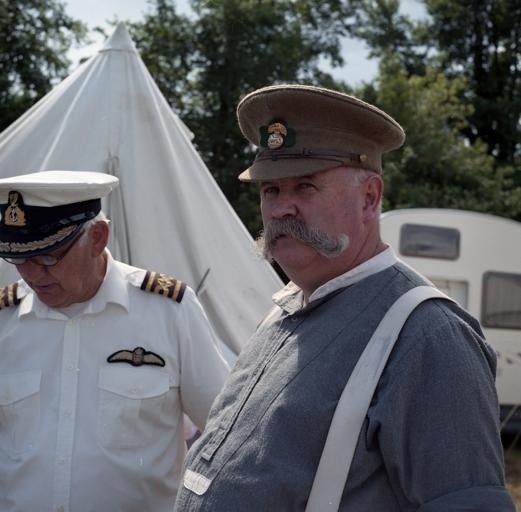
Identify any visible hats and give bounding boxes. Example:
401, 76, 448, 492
236, 85, 407, 183
0, 170, 120, 259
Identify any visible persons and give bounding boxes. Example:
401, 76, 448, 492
0, 166, 229, 511
175, 86, 516, 511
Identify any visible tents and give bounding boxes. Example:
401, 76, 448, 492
0, 21, 290, 448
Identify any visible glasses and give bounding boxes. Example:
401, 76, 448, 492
4, 228, 86, 267
